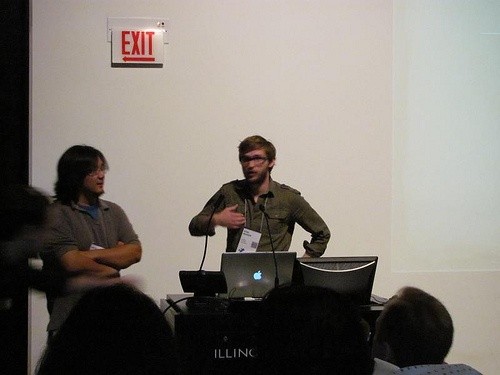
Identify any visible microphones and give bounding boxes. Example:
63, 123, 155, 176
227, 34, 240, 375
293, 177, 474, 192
258, 203, 279, 299
186, 193, 225, 310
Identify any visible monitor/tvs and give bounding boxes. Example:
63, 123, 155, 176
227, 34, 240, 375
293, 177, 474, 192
292, 256, 378, 305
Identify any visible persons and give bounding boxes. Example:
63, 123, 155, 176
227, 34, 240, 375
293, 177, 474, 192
38, 145, 141, 342
189, 136, 331, 258
0, 185, 119, 375
35, 282, 483, 375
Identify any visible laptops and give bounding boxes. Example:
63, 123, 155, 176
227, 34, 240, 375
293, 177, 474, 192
219, 252, 297, 299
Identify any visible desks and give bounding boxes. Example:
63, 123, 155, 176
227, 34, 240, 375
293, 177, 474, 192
159, 293, 390, 375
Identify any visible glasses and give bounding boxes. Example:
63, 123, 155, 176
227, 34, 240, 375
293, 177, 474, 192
240, 157, 267, 167
